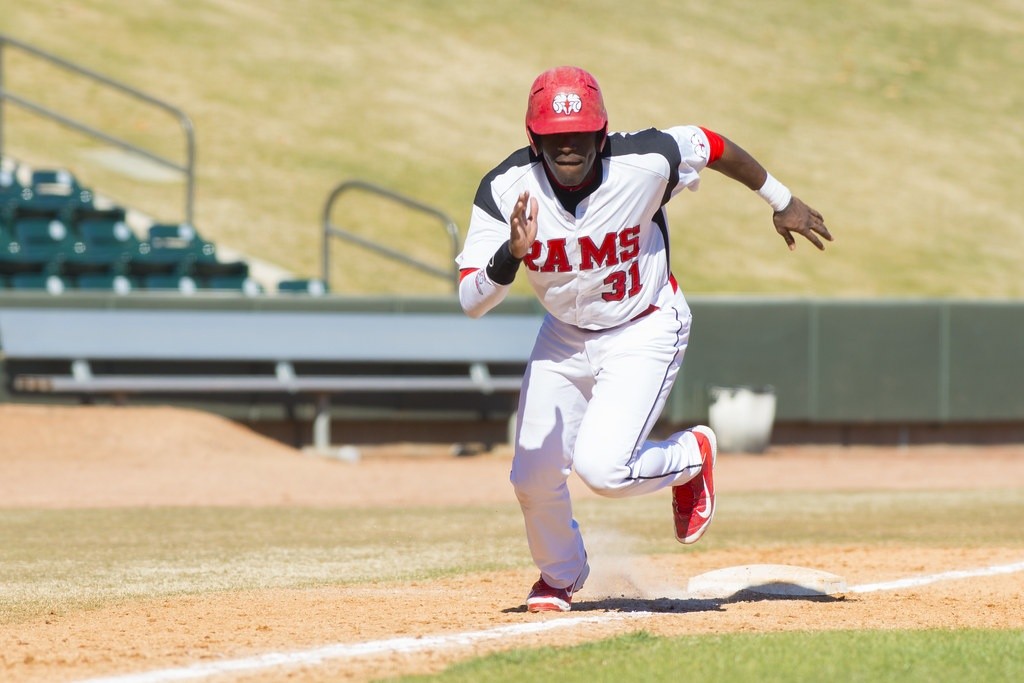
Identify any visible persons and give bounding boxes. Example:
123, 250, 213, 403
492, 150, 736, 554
455, 65, 834, 613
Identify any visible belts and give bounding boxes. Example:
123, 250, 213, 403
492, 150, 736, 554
629, 272, 677, 322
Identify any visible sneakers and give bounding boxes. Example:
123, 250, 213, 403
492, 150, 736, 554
526, 550, 590, 612
672, 424, 717, 544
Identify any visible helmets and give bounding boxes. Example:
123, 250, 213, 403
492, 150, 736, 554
525, 66, 608, 157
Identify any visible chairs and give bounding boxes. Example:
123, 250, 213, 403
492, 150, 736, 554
0, 166, 332, 295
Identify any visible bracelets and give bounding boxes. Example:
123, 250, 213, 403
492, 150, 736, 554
752, 171, 791, 212
486, 239, 522, 285
